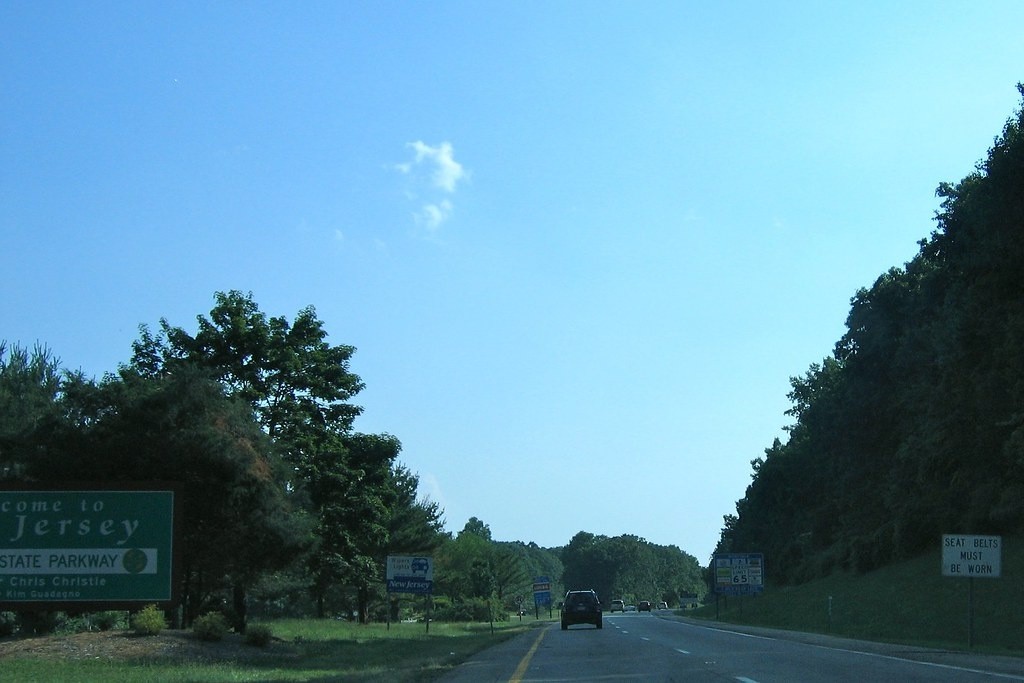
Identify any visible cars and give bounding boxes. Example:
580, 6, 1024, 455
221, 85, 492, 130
657, 602, 668, 610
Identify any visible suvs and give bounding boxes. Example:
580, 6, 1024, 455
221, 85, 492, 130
638, 601, 651, 612
559, 589, 603, 630
611, 600, 625, 613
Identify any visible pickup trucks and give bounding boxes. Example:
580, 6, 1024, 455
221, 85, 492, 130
625, 604, 636, 611
